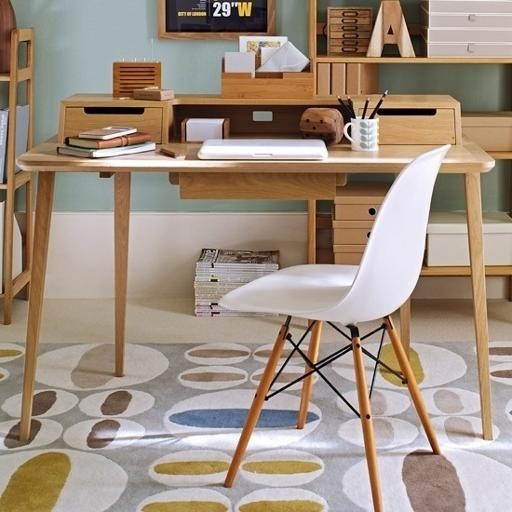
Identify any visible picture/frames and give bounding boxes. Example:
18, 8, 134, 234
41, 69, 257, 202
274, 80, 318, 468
158, 0, 276, 41
239, 36, 288, 72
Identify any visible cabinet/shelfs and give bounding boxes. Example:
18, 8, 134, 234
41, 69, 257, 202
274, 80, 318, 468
0, 32, 32, 325
308, 0, 512, 332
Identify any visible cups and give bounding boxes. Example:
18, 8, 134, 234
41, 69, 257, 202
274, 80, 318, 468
344, 116, 380, 151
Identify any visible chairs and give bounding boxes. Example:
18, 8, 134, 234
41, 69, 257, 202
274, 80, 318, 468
15, 92, 496, 441
217, 144, 453, 511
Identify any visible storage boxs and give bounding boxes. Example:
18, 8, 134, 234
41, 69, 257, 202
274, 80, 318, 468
332, 221, 375, 245
333, 245, 366, 264
461, 112, 512, 152
422, 0, 512, 58
334, 182, 388, 220
427, 212, 511, 267
327, 6, 373, 57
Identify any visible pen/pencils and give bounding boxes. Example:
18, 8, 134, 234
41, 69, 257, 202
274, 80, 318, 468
369, 89, 388, 119
336, 92, 354, 119
361, 97, 369, 118
346, 93, 356, 119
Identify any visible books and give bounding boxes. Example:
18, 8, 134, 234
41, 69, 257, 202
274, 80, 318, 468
55, 143, 158, 159
63, 132, 154, 149
0, 105, 30, 184
194, 248, 280, 319
78, 126, 140, 140
